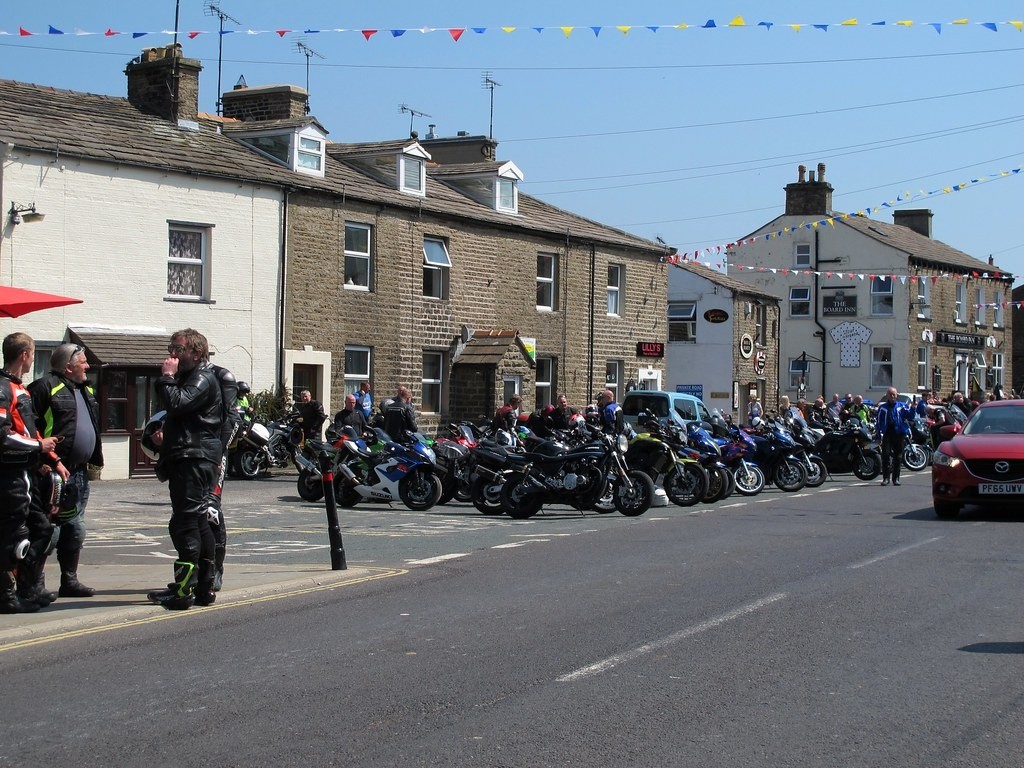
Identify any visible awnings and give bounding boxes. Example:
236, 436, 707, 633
0, 286, 84, 319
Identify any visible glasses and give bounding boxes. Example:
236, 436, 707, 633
167, 345, 187, 355
68, 345, 82, 364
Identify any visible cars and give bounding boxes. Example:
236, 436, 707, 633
930, 399, 1024, 520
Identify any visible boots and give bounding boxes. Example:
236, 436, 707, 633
146, 558, 216, 610
214, 547, 226, 591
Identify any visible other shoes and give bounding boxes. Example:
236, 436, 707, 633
0, 573, 50, 613
881, 476, 890, 486
893, 476, 901, 485
55, 574, 95, 597
32, 572, 57, 602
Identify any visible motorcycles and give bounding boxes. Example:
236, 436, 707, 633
235, 412, 302, 478
296, 393, 934, 520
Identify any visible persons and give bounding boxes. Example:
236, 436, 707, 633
226, 380, 1021, 486
0, 332, 70, 615
145, 328, 226, 607
200, 358, 241, 589
25, 342, 104, 598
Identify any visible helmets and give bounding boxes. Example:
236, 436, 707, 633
569, 413, 586, 429
140, 410, 167, 461
237, 381, 250, 393
542, 404, 555, 416
594, 392, 604, 407
838, 410, 849, 421
379, 399, 395, 414
752, 417, 765, 430
495, 427, 516, 448
588, 404, 595, 411
